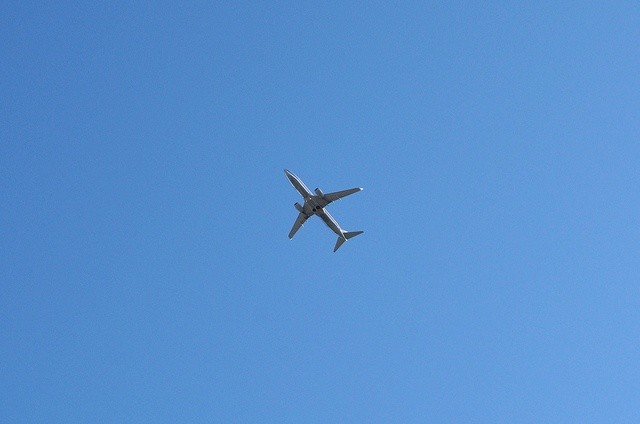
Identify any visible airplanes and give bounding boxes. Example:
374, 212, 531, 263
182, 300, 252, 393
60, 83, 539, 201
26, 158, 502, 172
283, 168, 363, 252
